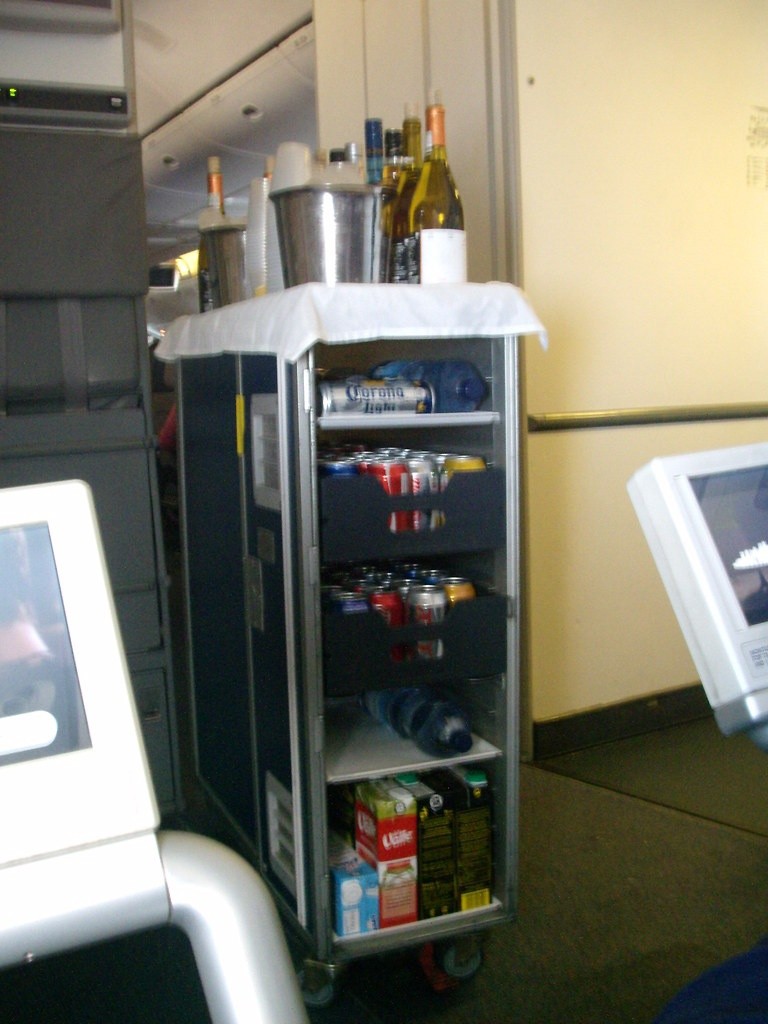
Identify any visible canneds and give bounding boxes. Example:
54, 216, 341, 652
314, 373, 489, 678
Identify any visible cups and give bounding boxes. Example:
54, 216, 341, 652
244, 176, 271, 295
263, 140, 357, 293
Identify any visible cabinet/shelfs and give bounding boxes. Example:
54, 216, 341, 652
152, 275, 552, 1014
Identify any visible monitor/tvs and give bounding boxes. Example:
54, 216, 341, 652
625, 441, 767, 707
0, 478, 159, 870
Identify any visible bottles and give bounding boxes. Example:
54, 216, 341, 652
391, 98, 422, 284
410, 90, 470, 286
364, 113, 384, 186
372, 357, 487, 412
318, 382, 436, 418
358, 686, 471, 758
378, 127, 407, 285
197, 156, 227, 314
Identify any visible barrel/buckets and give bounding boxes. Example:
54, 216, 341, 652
270, 182, 378, 290
203, 224, 250, 309
